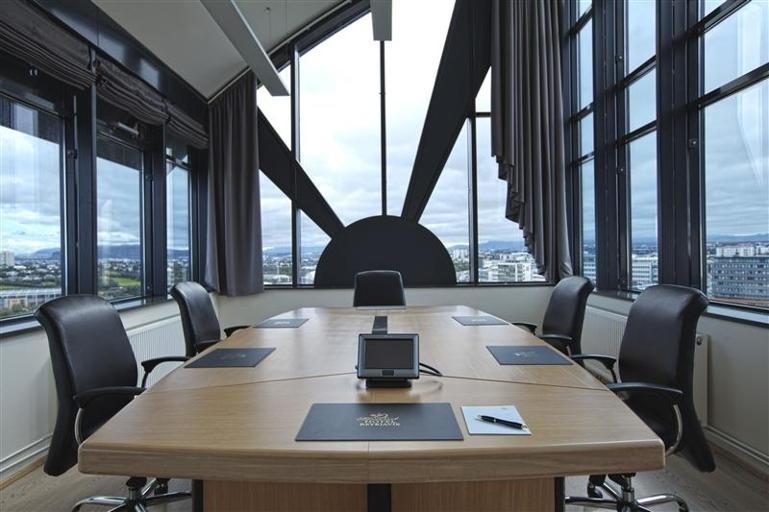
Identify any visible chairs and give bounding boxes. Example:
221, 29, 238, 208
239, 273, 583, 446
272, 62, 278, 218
564, 284, 716, 511
510, 274, 593, 366
35, 292, 194, 510
353, 269, 406, 307
170, 280, 249, 359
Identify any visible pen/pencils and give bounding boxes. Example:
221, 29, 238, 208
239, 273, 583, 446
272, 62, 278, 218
478, 414, 524, 429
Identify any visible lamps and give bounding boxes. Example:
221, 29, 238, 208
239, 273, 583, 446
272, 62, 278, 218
371, 1, 392, 40
199, 1, 289, 97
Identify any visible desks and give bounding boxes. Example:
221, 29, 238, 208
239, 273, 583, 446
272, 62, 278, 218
77, 305, 666, 511
